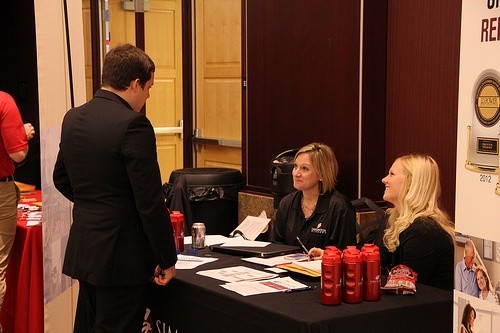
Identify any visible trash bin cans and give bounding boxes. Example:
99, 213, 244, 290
271, 149, 298, 209
162, 168, 246, 236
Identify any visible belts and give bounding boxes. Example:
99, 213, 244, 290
0, 175, 14, 182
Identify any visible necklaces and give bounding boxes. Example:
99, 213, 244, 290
301, 197, 316, 220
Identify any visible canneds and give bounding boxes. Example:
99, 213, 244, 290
191, 223, 205, 249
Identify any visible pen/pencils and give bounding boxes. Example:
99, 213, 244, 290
176, 249, 211, 257
297, 236, 313, 258
19, 210, 42, 221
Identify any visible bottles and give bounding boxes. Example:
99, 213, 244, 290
319, 243, 381, 305
170, 211, 185, 253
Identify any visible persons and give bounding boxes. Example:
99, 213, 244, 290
460, 304, 476, 333
272, 142, 356, 252
0, 90, 35, 333
309, 153, 454, 290
456, 241, 496, 303
53, 43, 178, 333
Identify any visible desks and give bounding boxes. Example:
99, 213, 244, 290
0, 189, 45, 333
142, 244, 453, 333
